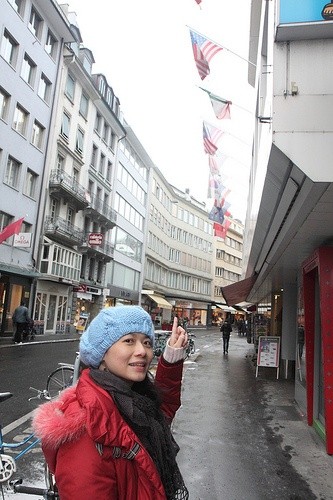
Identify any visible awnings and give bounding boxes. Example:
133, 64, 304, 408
146, 294, 173, 310
216, 275, 257, 314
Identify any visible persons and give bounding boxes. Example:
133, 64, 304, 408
11, 302, 31, 344
172, 314, 246, 354
31, 304, 190, 500
298, 323, 305, 360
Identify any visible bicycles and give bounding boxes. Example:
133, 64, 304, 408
154, 330, 197, 357
0, 387, 62, 500
46, 351, 155, 399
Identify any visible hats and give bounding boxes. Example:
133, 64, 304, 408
79, 305, 154, 369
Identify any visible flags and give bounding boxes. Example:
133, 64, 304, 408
190, 0, 231, 240
0, 217, 25, 245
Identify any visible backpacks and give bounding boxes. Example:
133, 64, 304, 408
223, 323, 231, 336
